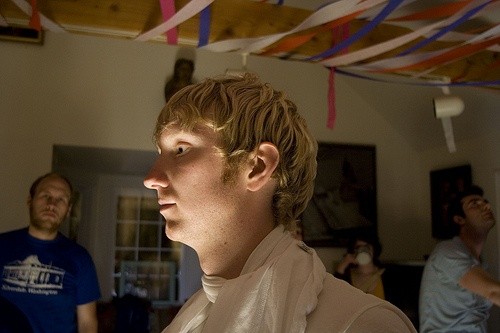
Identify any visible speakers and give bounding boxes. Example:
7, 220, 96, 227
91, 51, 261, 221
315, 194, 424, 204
430, 163, 473, 240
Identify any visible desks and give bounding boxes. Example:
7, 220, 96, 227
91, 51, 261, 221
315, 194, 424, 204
390, 257, 425, 272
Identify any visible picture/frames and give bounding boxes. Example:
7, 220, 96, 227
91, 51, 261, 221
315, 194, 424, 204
427, 164, 475, 240
294, 140, 383, 251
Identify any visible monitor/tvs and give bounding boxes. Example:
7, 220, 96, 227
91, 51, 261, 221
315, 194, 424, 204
298, 141, 378, 247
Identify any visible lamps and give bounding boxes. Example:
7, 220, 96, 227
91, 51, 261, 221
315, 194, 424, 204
430, 82, 465, 124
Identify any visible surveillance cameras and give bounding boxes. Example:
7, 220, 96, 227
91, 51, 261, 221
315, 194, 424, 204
433, 96, 465, 119
174, 47, 195, 80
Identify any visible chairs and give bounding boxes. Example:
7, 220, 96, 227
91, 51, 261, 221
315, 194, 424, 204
97, 293, 158, 333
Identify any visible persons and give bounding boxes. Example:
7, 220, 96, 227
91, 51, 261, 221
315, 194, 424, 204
0, 172, 102, 333
419, 186, 500, 333
333, 233, 398, 305
143, 73, 419, 333
